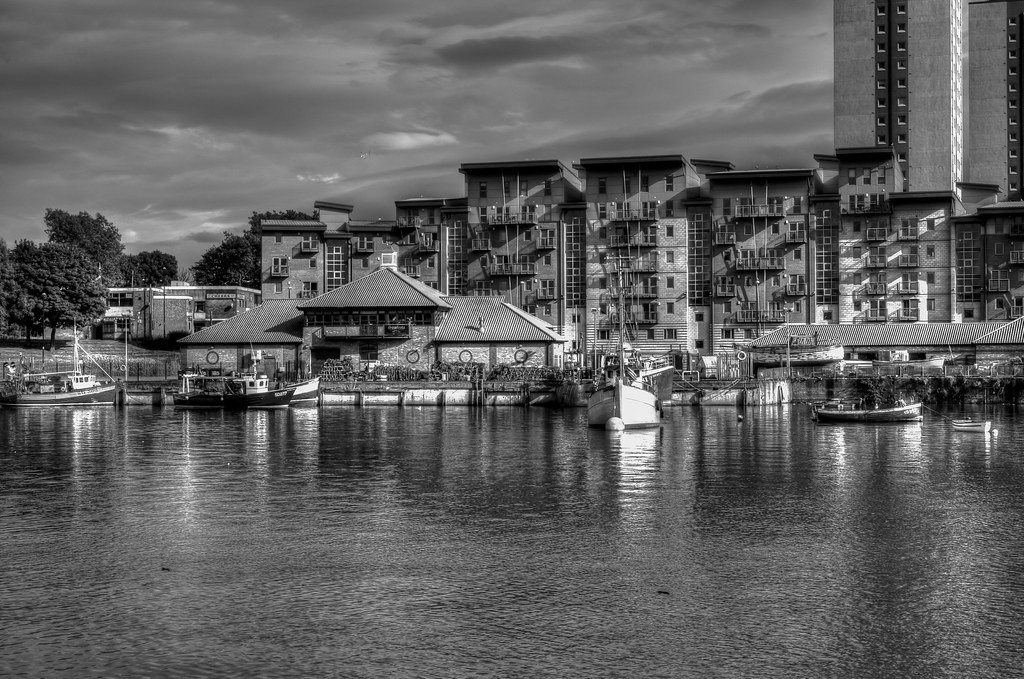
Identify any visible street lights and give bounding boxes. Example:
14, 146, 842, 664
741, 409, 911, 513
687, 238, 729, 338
42, 293, 47, 370
591, 307, 597, 375
783, 307, 795, 380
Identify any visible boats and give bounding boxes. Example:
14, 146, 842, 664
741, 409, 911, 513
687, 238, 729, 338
173, 345, 295, 409
555, 355, 674, 408
181, 356, 320, 406
815, 401, 923, 422
0, 319, 126, 407
951, 418, 991, 433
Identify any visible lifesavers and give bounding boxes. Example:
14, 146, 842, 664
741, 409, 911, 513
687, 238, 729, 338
737, 351, 747, 360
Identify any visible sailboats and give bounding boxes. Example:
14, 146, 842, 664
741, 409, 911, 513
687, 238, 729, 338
586, 256, 661, 431
